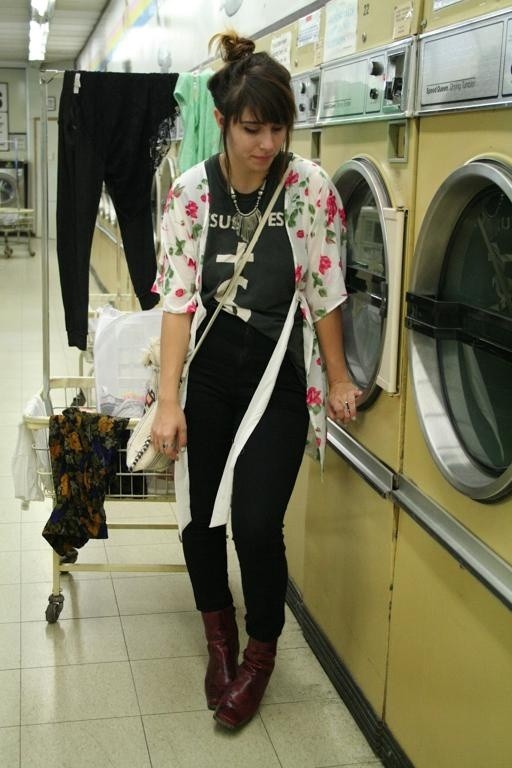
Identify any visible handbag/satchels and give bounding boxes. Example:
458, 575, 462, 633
127, 379, 174, 474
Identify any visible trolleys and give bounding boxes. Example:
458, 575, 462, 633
21, 291, 191, 624
0, 134, 37, 261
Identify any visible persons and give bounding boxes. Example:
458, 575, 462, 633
145, 25, 364, 735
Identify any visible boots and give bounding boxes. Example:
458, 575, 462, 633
213, 635, 274, 729
203, 606, 239, 710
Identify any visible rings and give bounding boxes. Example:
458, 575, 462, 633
344, 401, 349, 406
164, 443, 177, 451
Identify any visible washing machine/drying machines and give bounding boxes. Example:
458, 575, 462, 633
0, 160, 27, 209
297, 0, 422, 756
379, 0, 512, 768
87, 0, 320, 622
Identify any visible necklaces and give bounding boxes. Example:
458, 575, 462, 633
224, 156, 267, 242
